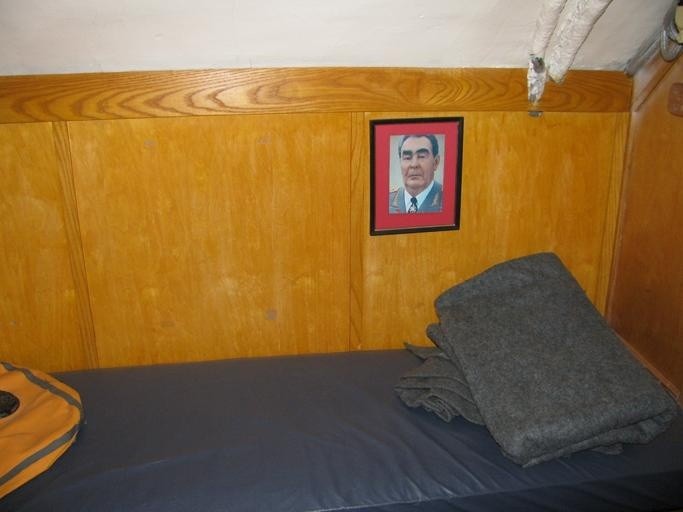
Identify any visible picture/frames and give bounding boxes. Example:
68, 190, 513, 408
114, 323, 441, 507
370, 117, 464, 236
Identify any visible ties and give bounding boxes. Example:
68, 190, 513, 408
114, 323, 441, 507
408, 198, 417, 214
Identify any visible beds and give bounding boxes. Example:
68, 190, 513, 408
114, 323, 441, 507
0, 346, 682, 511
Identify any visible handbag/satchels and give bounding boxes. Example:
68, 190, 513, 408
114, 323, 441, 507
0, 362, 83, 499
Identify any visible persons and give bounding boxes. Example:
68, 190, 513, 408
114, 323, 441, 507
389, 134, 443, 213
